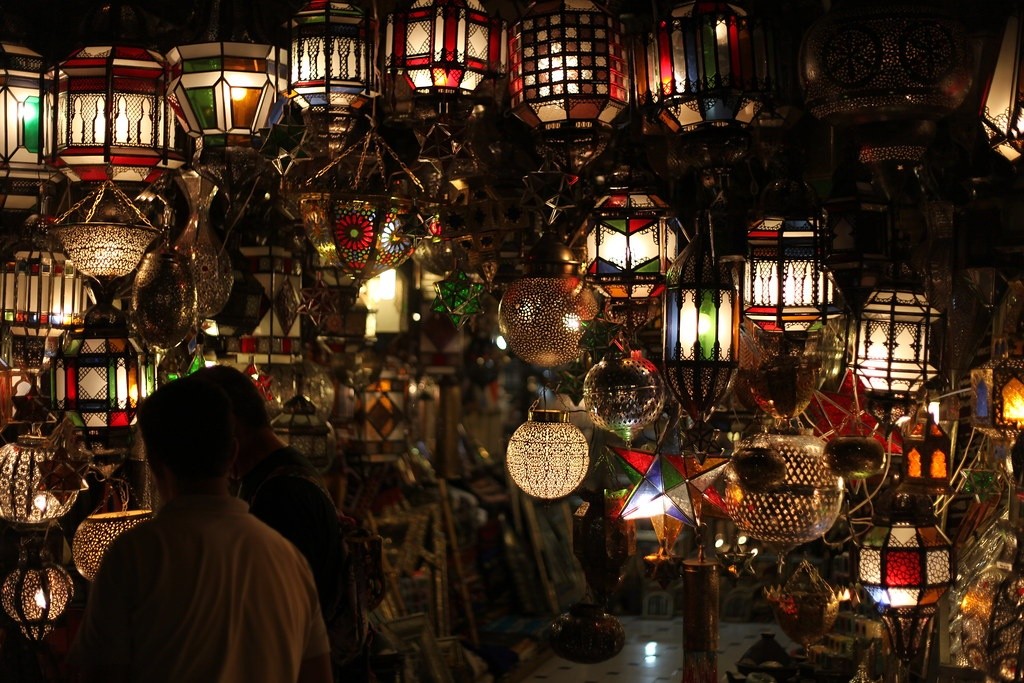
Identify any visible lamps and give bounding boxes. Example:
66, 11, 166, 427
0, 0, 1024, 683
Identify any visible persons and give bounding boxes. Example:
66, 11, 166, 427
58, 376, 328, 683
190, 365, 369, 683
